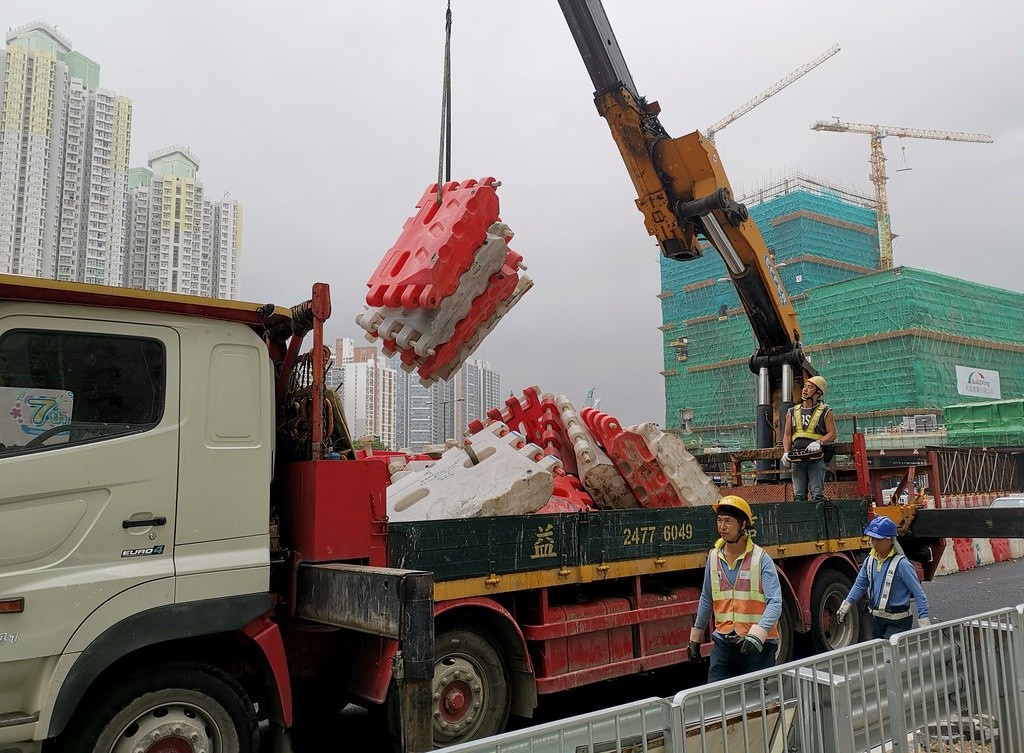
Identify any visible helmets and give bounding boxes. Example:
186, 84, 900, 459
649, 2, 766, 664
863, 517, 898, 538
712, 494, 752, 527
806, 376, 827, 395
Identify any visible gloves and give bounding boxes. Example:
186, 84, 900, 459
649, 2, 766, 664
836, 598, 854, 626
735, 634, 764, 658
686, 639, 705, 665
805, 440, 820, 453
781, 452, 791, 469
917, 617, 931, 629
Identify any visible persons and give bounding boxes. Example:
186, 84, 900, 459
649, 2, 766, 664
688, 496, 782, 707
782, 376, 837, 502
836, 516, 931, 641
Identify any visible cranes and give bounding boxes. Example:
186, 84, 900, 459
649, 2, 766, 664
808, 116, 995, 270
701, 42, 841, 150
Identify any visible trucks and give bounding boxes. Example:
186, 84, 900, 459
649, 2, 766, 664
1, 282, 879, 753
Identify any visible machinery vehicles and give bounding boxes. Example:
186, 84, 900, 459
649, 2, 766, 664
439, 0, 927, 544
